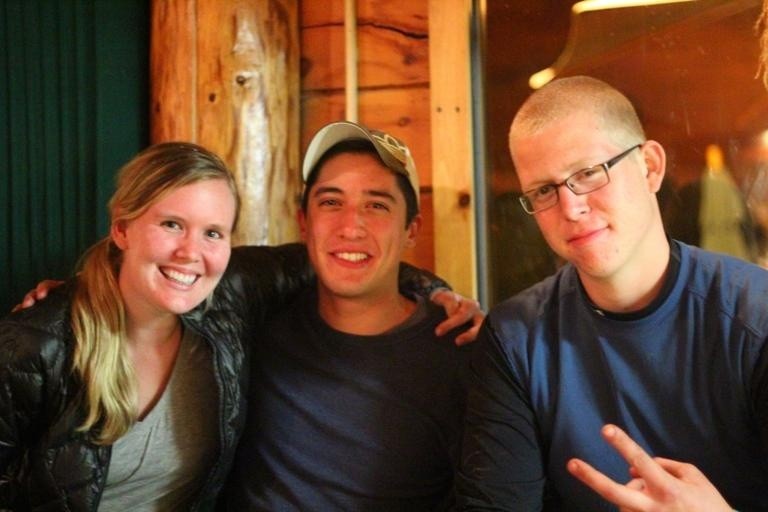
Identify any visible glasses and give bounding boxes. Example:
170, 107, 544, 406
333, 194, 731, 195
515, 144, 650, 219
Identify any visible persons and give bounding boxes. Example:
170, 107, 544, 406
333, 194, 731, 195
448, 77, 768, 512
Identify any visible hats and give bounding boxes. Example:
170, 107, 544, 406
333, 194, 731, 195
301, 120, 422, 217
